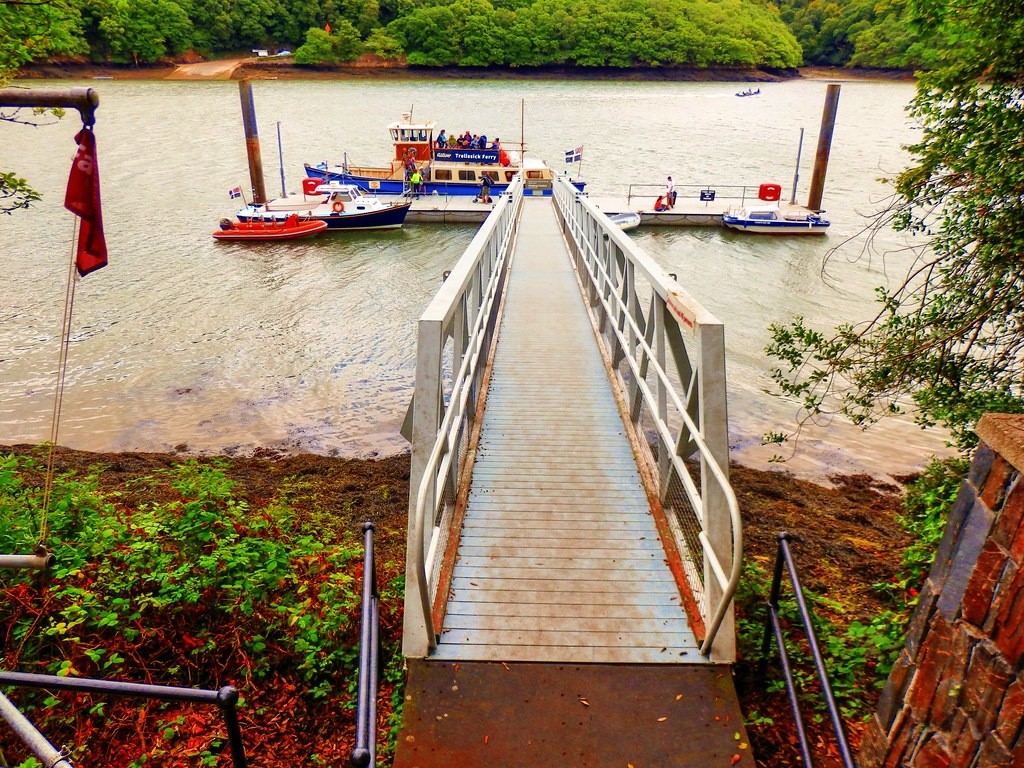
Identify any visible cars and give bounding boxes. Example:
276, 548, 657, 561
278, 51, 290, 56
277, 48, 291, 53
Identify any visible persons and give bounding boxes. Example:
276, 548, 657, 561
402, 145, 428, 200
436, 129, 500, 150
654, 176, 674, 211
478, 171, 494, 203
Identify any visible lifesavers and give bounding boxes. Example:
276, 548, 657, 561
332, 201, 344, 213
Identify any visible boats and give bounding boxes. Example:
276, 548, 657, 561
304, 98, 586, 197
212, 213, 328, 241
594, 212, 641, 236
721, 127, 830, 235
235, 178, 412, 230
743, 88, 759, 95
736, 92, 745, 96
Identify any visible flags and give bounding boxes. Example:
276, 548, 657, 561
565, 146, 583, 162
228, 186, 242, 199
